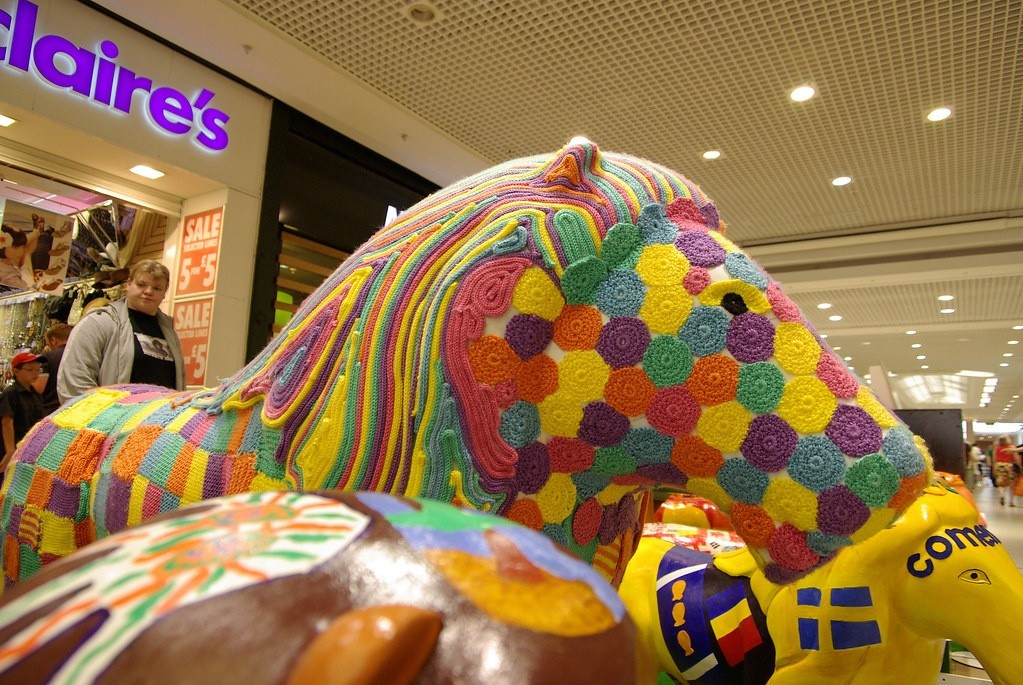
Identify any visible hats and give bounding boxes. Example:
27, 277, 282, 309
12, 353, 41, 367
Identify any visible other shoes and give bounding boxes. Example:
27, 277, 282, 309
1000, 497, 1004, 505
1009, 504, 1015, 507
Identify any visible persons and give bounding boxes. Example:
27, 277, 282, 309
0, 214, 72, 291
57, 259, 187, 408
0, 325, 71, 492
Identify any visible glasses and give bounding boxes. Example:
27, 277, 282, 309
22, 368, 43, 373
